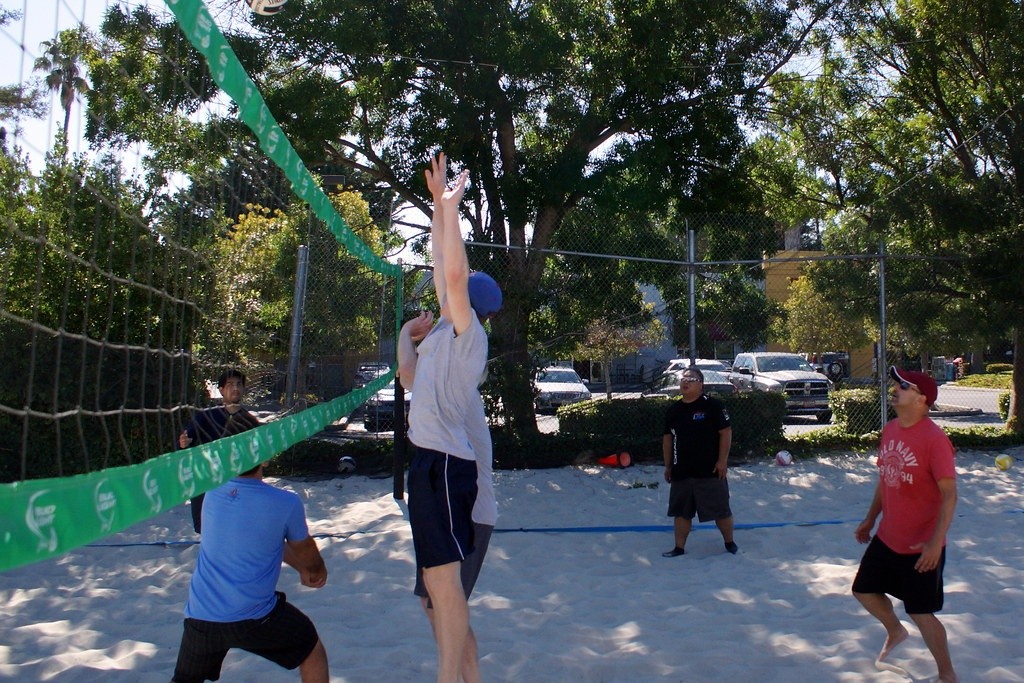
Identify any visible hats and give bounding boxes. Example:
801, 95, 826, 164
468, 271, 503, 318
890, 365, 938, 406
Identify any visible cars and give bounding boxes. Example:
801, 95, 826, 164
663, 358, 730, 372
642, 370, 738, 399
356, 363, 412, 430
530, 368, 592, 414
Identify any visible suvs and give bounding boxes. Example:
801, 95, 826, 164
799, 353, 844, 390
732, 352, 836, 424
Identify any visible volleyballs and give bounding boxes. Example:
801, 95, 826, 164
245, 0, 288, 17
774, 450, 793, 466
994, 454, 1013, 471
337, 456, 357, 474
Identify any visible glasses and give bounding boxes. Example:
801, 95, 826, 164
896, 378, 909, 390
681, 376, 701, 382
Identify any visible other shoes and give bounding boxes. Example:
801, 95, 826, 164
725, 542, 739, 554
662, 547, 685, 557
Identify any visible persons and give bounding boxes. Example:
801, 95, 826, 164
852, 365, 960, 683
393, 150, 504, 683
662, 367, 745, 557
178, 369, 270, 535
170, 411, 330, 683
